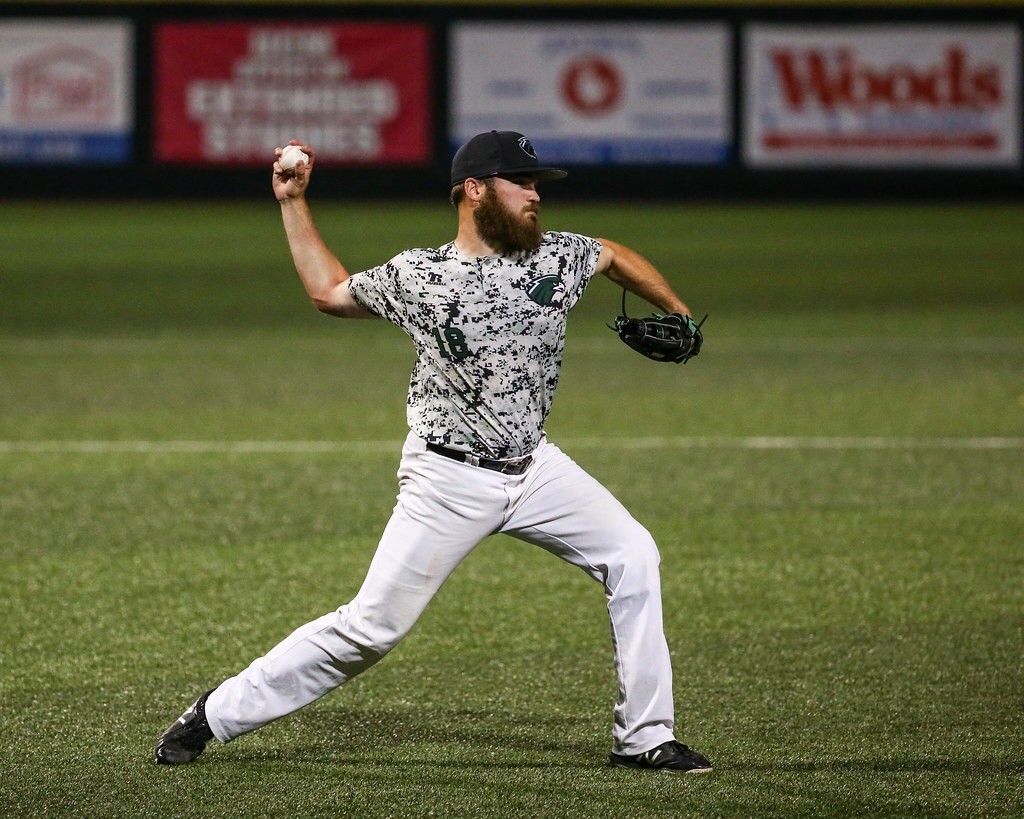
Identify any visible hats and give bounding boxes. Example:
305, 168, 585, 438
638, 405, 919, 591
450, 132, 568, 186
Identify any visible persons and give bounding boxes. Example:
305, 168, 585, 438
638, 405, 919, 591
156, 130, 714, 775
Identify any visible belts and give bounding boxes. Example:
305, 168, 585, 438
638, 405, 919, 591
425, 440, 533, 475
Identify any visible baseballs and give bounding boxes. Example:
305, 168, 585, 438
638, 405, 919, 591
278, 144, 309, 173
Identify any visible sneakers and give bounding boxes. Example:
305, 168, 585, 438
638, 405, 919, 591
611, 741, 714, 774
152, 688, 214, 767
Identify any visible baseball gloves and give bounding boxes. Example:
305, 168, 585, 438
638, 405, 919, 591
614, 309, 704, 364
